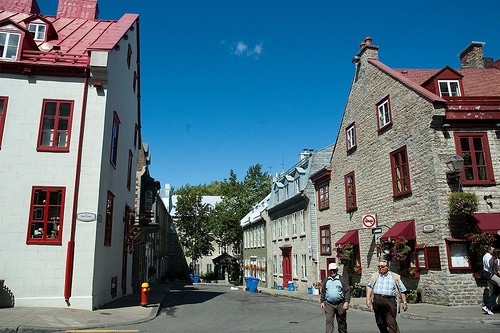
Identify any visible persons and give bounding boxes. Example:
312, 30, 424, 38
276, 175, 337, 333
366, 260, 408, 333
481, 246, 500, 315
320, 263, 351, 333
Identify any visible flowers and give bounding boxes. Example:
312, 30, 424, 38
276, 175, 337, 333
336, 243, 353, 261
448, 191, 479, 215
466, 233, 497, 254
384, 237, 411, 262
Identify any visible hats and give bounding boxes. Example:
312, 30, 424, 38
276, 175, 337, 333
328, 263, 338, 270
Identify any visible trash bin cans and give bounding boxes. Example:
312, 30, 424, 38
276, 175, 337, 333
194, 274, 199, 283
245, 277, 254, 291
249, 278, 260, 293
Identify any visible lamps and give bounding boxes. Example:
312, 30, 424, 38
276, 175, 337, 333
442, 124, 450, 130
446, 155, 464, 178
53, 45, 60, 53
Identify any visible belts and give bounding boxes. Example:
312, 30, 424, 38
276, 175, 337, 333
327, 299, 343, 305
374, 294, 396, 299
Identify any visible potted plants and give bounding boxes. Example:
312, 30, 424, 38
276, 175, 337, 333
400, 270, 420, 278
347, 266, 361, 274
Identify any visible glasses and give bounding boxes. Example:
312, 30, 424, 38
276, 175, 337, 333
377, 265, 385, 268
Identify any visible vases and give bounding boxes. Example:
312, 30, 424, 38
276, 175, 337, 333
341, 259, 347, 264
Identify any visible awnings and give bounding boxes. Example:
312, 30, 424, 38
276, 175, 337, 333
335, 230, 359, 247
473, 213, 500, 234
381, 219, 416, 240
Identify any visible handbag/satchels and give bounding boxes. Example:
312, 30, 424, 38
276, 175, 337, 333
480, 270, 491, 280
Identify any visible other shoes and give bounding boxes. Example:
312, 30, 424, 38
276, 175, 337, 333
482, 306, 493, 315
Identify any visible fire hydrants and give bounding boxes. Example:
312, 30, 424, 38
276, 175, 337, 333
139, 282, 151, 307
